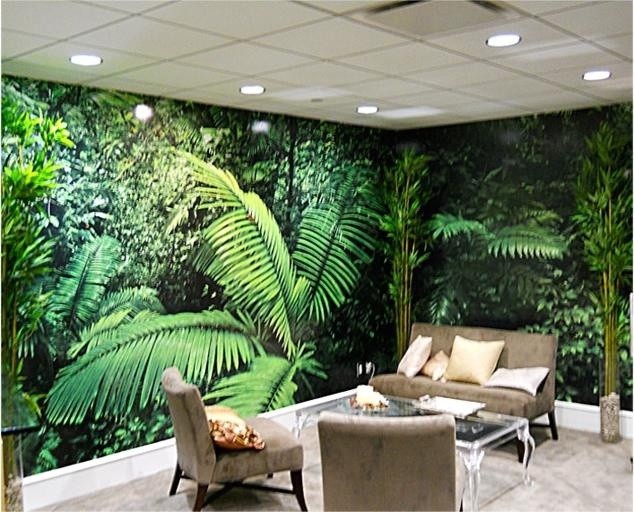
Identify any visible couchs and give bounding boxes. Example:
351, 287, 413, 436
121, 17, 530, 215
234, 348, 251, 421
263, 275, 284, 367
369, 323, 558, 462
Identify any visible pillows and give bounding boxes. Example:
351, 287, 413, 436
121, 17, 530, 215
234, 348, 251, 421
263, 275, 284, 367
443, 334, 506, 385
484, 367, 551, 397
397, 335, 432, 378
204, 405, 266, 450
422, 351, 449, 381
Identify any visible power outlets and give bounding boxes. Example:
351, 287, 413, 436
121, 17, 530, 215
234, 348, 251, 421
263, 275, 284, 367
356, 361, 371, 378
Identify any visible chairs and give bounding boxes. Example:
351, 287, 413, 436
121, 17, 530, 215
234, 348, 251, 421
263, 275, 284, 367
318, 410, 466, 512
161, 366, 309, 512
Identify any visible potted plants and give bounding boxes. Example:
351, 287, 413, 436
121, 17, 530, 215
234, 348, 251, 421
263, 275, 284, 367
356, 144, 434, 370
1, 97, 73, 512
573, 120, 633, 443
